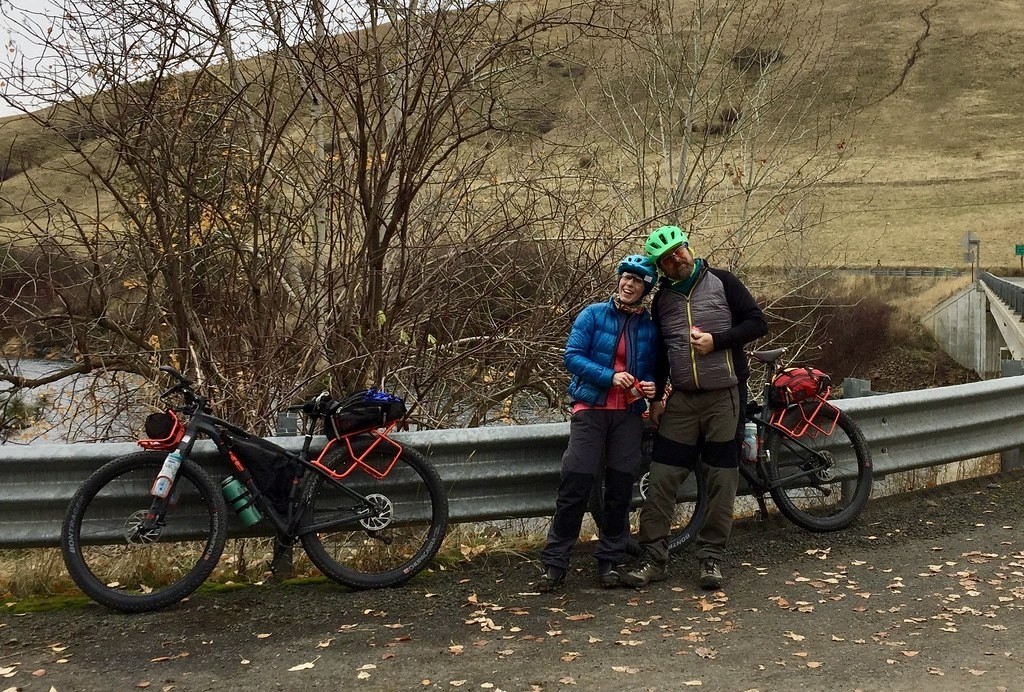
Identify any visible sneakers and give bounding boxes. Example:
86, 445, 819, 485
622, 559, 669, 588
699, 559, 724, 587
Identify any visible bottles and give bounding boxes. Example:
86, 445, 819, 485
744, 420, 758, 459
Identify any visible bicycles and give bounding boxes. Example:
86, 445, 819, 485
589, 347, 873, 558
59, 363, 450, 612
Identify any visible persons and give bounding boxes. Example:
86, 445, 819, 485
627, 226, 770, 590
534, 255, 656, 590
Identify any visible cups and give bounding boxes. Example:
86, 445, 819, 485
219, 474, 262, 527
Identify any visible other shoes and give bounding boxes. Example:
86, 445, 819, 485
537, 568, 566, 590
598, 562, 621, 588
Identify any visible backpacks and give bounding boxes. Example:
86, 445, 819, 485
770, 367, 831, 410
325, 388, 407, 440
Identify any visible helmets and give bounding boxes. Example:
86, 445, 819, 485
644, 224, 689, 266
617, 255, 659, 285
